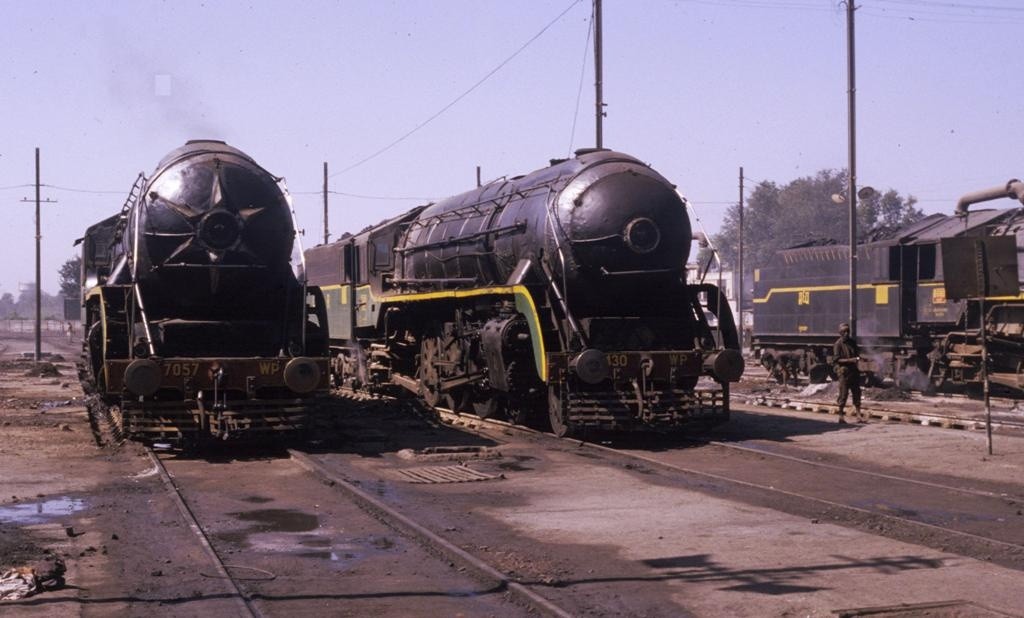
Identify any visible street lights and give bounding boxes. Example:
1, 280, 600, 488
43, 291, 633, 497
831, 182, 875, 338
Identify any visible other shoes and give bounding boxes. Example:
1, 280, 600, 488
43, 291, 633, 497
857, 418, 868, 424
838, 418, 846, 424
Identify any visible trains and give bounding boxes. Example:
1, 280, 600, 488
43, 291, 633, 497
749, 177, 1024, 399
297, 145, 745, 439
72, 141, 344, 441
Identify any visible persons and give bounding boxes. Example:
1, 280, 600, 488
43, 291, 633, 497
761, 352, 789, 387
66, 322, 75, 345
833, 323, 868, 423
